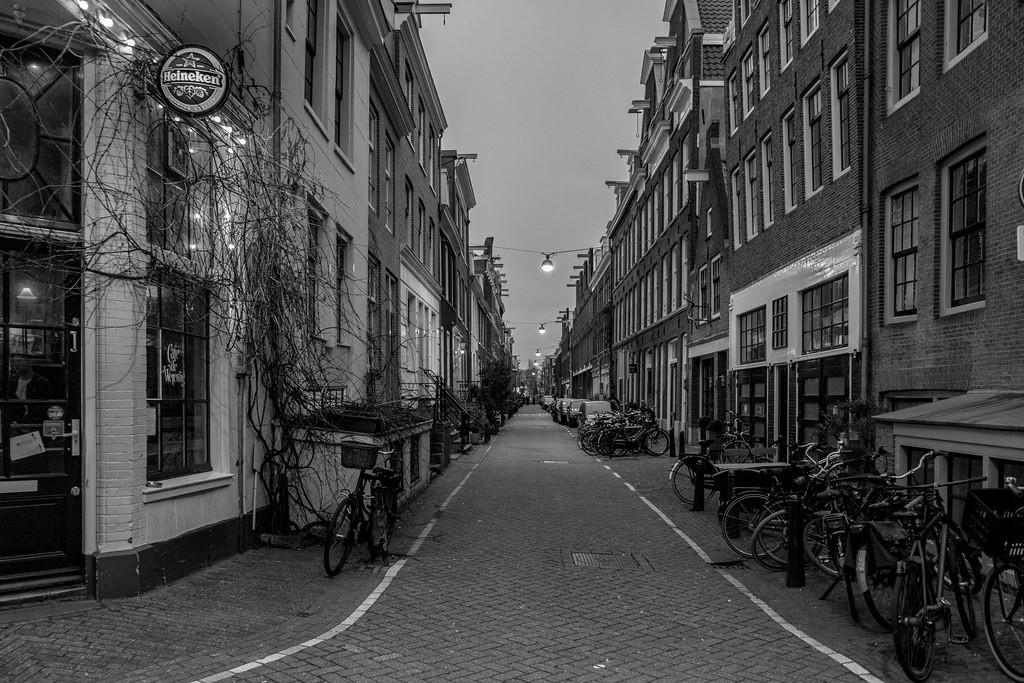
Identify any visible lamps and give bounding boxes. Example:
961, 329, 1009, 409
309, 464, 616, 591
17, 281, 38, 300
719, 374, 725, 386
540, 255, 555, 272
536, 349, 540, 357
539, 324, 545, 333
707, 377, 713, 391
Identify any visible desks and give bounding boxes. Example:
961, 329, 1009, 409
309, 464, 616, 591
715, 462, 792, 471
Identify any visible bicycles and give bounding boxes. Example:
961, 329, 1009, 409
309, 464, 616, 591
958, 476, 1024, 683
576, 400, 989, 683
323, 440, 403, 577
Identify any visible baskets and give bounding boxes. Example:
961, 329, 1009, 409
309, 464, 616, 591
340, 441, 379, 470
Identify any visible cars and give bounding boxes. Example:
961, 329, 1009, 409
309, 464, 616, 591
566, 398, 591, 428
576, 400, 612, 429
540, 394, 572, 425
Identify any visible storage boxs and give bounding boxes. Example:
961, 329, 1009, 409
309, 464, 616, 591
961, 487, 1024, 560
341, 410, 380, 433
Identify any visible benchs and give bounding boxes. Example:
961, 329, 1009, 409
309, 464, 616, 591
724, 448, 776, 463
